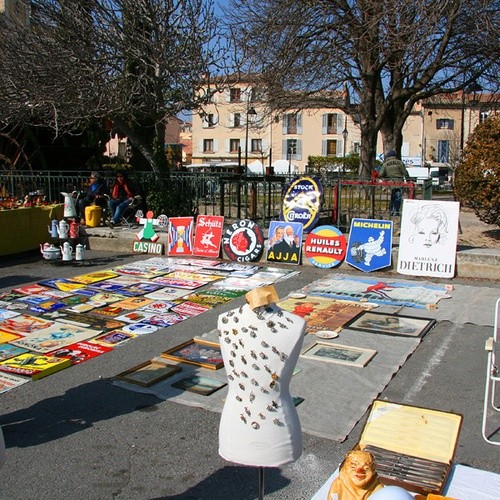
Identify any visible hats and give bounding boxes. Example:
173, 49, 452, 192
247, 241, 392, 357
387, 151, 396, 157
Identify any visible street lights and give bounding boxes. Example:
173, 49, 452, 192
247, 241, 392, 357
244, 90, 259, 194
341, 115, 349, 174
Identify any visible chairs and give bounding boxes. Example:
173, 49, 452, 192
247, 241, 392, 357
482, 298, 500, 445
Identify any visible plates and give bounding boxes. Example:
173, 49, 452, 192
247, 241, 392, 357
287, 293, 306, 298
316, 331, 338, 338
361, 303, 380, 308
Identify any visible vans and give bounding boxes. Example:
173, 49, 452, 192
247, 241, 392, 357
183, 164, 253, 175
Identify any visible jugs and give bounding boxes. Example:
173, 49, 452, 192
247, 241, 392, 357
60, 241, 73, 261
47, 218, 59, 238
59, 190, 80, 217
40, 243, 50, 260
76, 243, 86, 260
70, 219, 80, 238
56, 219, 69, 239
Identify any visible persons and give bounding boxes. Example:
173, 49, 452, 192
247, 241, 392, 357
327, 450, 382, 500
217, 304, 305, 468
173, 162, 187, 193
105, 171, 141, 229
76, 171, 106, 224
379, 151, 412, 216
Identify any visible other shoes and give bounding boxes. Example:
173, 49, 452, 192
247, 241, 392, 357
105, 220, 114, 229
390, 211, 400, 216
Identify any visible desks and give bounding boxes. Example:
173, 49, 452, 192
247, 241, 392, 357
0, 199, 67, 258
311, 444, 500, 500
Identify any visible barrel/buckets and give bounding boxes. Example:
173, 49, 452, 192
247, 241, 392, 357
85, 205, 102, 227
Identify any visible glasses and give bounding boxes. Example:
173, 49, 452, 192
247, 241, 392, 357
116, 176, 122, 179
88, 177, 93, 179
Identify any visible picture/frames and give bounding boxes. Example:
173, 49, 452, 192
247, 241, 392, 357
118, 309, 436, 395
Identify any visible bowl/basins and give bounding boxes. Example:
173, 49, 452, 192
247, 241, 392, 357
43, 244, 60, 259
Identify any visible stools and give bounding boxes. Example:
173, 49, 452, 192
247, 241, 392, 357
122, 207, 137, 227
